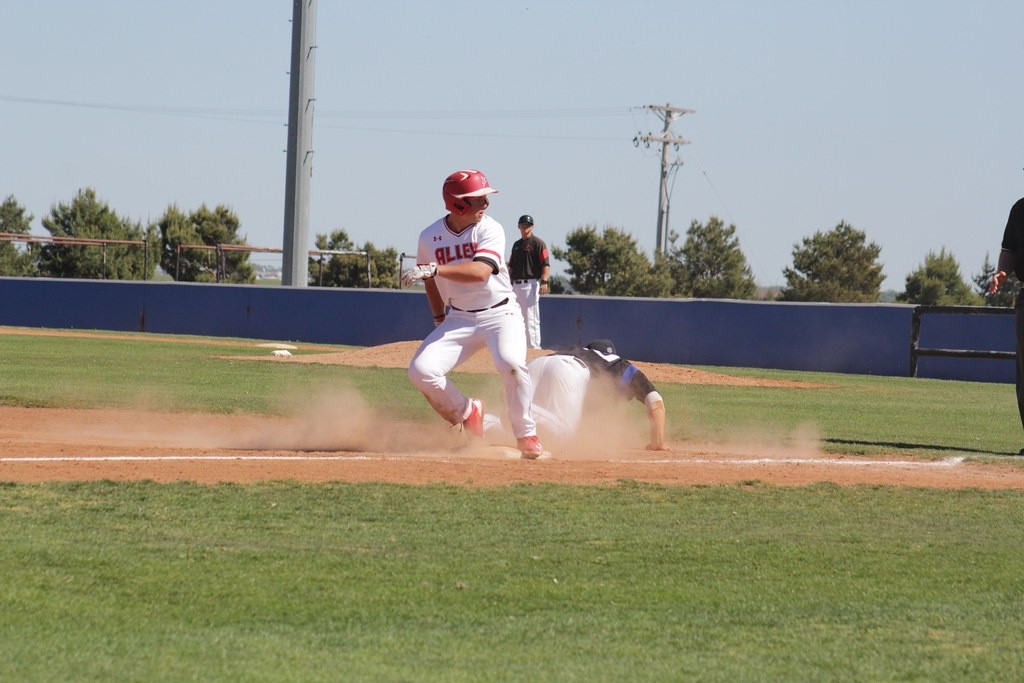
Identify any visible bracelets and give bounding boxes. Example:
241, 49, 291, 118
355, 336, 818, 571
433, 314, 445, 322
541, 280, 548, 284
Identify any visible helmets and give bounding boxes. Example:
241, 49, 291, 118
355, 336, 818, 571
443, 170, 499, 215
518, 215, 533, 225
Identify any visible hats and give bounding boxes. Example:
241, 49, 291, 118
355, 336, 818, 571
584, 338, 616, 356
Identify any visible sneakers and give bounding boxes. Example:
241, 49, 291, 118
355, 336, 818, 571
517, 436, 543, 459
463, 399, 484, 439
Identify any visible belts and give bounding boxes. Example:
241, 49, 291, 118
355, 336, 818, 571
451, 298, 509, 312
515, 279, 538, 284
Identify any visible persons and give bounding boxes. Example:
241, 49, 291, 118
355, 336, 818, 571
989, 198, 1024, 455
507, 215, 550, 350
401, 170, 544, 460
483, 339, 671, 451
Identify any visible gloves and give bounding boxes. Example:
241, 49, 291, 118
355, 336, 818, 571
401, 262, 437, 288
